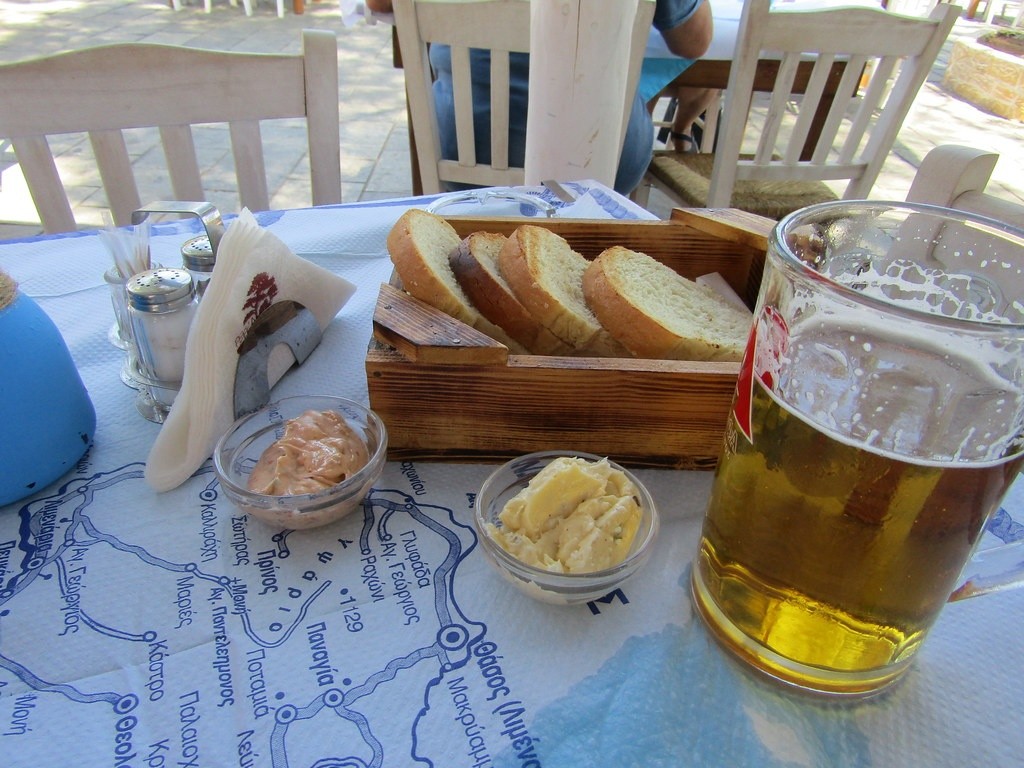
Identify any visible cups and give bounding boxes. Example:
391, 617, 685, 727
691, 201, 1024, 701
104, 259, 163, 340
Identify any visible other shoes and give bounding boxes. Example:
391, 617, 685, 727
670, 128, 699, 154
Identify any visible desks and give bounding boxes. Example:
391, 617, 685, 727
382, 0, 868, 186
0, 180, 1024, 767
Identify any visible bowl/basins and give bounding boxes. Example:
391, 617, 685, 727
213, 394, 389, 529
476, 450, 659, 604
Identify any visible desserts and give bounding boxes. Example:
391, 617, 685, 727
484, 453, 642, 605
240, 409, 374, 531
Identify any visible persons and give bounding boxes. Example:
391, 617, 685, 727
648, 86, 719, 153
365, 0, 712, 194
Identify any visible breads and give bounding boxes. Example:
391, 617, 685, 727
385, 209, 752, 362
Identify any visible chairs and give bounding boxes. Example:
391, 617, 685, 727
633, 2, 965, 215
0, 30, 341, 243
391, 0, 657, 196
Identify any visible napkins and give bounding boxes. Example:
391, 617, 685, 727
143, 206, 359, 492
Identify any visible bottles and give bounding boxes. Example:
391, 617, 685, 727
181, 235, 219, 298
127, 268, 202, 411
